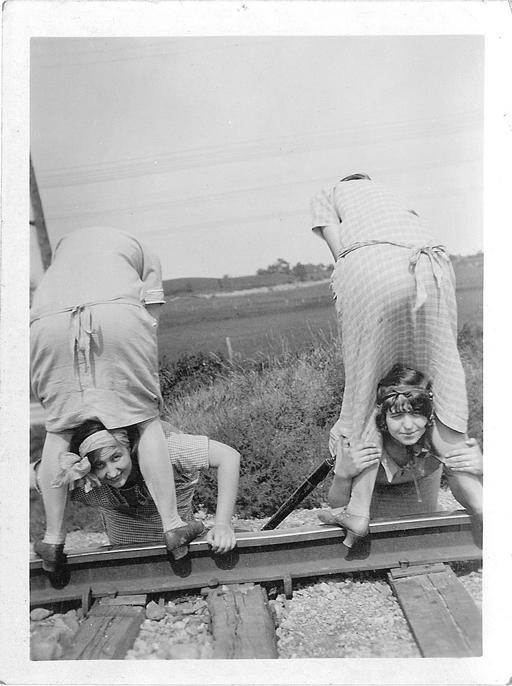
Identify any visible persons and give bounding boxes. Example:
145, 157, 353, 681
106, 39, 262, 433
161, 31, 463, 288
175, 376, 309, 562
29, 414, 245, 557
29, 218, 207, 572
306, 169, 484, 546
322, 362, 484, 521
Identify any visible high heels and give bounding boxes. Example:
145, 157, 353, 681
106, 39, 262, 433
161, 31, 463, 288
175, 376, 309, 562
33, 539, 64, 572
318, 511, 370, 547
164, 520, 204, 562
